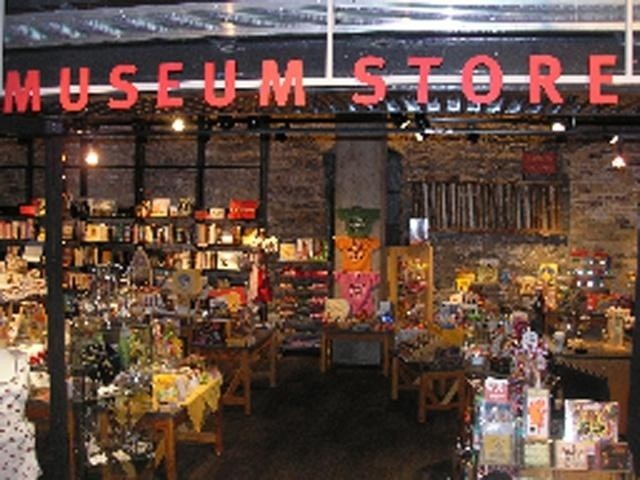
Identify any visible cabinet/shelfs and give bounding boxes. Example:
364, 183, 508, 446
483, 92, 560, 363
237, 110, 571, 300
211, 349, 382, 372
384, 245, 433, 326
1, 211, 332, 353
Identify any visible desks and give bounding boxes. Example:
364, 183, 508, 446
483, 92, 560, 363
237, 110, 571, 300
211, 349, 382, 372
94, 324, 277, 480
386, 344, 469, 426
324, 320, 391, 376
